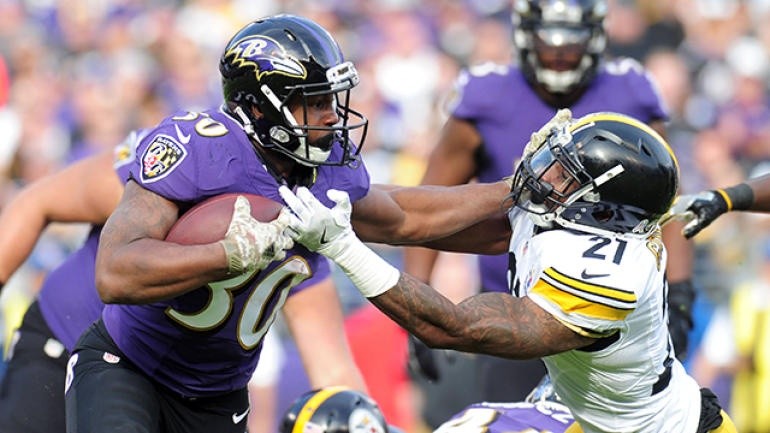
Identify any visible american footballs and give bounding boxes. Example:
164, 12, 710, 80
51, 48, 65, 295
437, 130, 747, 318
166, 194, 283, 246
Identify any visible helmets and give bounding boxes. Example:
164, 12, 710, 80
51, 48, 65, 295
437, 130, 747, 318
511, 112, 681, 238
279, 386, 389, 433
219, 14, 367, 170
511, 0, 610, 105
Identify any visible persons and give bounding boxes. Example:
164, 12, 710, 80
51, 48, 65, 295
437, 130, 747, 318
668, 170, 770, 240
0, 124, 512, 433
277, 109, 738, 433
402, 1, 699, 429
66, 14, 581, 433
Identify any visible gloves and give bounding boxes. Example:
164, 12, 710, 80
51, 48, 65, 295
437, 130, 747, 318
407, 332, 457, 385
670, 188, 732, 240
667, 280, 697, 361
279, 185, 401, 297
221, 196, 294, 273
